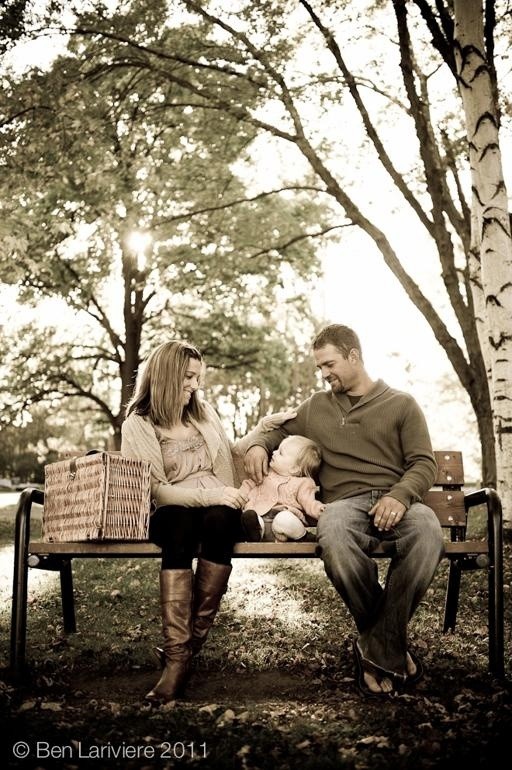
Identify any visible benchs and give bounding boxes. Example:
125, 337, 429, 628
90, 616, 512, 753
9, 449, 505, 684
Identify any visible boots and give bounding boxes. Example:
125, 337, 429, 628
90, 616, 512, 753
145, 557, 234, 702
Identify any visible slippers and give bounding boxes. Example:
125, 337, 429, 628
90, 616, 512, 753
352, 635, 425, 698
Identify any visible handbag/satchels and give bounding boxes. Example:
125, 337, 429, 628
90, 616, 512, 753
39, 451, 153, 544
